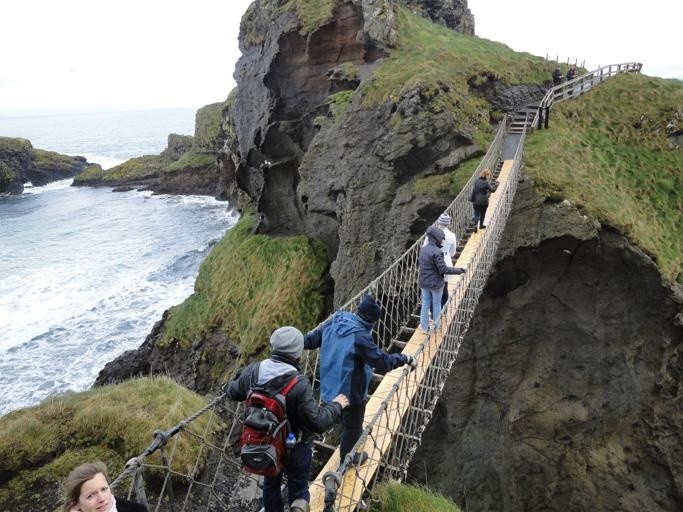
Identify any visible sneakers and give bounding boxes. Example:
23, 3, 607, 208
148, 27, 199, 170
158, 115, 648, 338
340, 451, 368, 468
434, 327, 440, 332
474, 226, 477, 233
422, 327, 430, 334
480, 226, 486, 228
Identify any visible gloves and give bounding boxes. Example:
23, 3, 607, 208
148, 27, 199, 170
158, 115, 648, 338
407, 355, 417, 371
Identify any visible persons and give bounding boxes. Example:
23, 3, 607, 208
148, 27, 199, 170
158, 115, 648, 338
468, 168, 498, 234
221, 325, 351, 511
423, 213, 458, 320
419, 228, 466, 335
551, 66, 563, 100
62, 461, 147, 511
565, 65, 578, 96
302, 296, 419, 465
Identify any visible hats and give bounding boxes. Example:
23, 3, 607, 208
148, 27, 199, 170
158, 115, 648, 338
358, 295, 381, 322
438, 213, 450, 225
270, 326, 305, 360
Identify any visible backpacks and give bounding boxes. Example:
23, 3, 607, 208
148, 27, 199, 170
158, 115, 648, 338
240, 363, 306, 477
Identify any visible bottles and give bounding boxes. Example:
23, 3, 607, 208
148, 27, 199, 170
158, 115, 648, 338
286, 434, 297, 448
